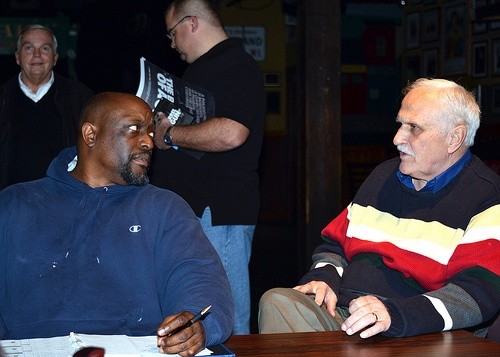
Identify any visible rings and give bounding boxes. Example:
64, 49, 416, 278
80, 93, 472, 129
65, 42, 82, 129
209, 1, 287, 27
372, 312, 379, 323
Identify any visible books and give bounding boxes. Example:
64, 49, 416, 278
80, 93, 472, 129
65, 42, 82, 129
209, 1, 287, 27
0, 332, 214, 357
136, 57, 217, 160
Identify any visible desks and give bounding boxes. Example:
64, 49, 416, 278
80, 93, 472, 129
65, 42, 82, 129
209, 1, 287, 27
223, 330, 500, 357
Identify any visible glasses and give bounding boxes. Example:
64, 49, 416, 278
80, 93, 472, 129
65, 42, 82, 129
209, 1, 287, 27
166, 16, 196, 40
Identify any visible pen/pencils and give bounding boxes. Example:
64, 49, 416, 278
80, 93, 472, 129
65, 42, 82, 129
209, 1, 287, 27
166, 305, 212, 338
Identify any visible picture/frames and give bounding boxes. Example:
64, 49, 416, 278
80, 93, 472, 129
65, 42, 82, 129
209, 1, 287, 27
403, 0, 500, 117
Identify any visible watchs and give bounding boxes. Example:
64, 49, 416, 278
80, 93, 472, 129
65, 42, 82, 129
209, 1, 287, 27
163, 126, 174, 147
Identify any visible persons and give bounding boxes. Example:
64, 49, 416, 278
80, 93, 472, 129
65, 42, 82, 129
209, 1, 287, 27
0, 93, 235, 357
0, 0, 268, 335
258, 79, 499, 343
